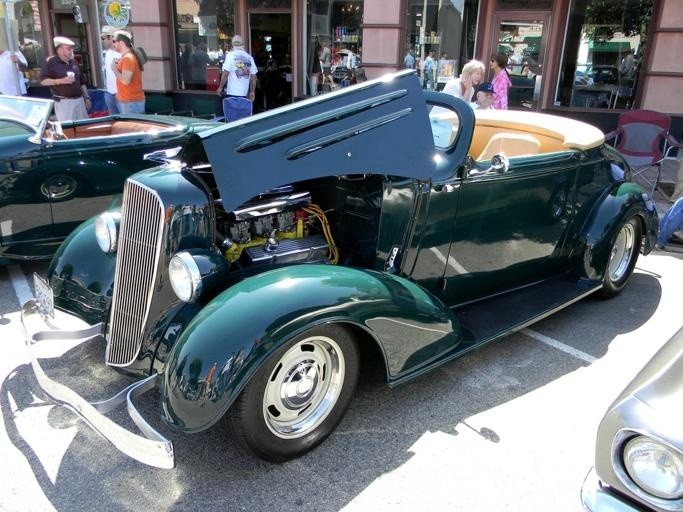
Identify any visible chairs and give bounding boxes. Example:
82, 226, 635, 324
476, 132, 540, 163
601, 109, 681, 204
146, 96, 253, 123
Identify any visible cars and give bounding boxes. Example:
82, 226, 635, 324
34, 72, 662, 461
578, 329, 682, 509
506, 65, 634, 109
2, 142, 171, 203
0, 93, 228, 275
375, 153, 596, 245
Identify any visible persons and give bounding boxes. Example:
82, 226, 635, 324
38, 36, 92, 120
488, 51, 512, 110
249, 34, 293, 115
163, 336, 262, 409
108, 29, 147, 114
177, 40, 230, 91
98, 25, 121, 116
472, 82, 500, 112
505, 44, 645, 109
214, 35, 259, 103
162, 199, 214, 257
308, 36, 457, 96
0, 49, 29, 98
640, 197, 682, 251
439, 58, 485, 105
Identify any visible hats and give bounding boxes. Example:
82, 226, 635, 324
130, 43, 148, 72
233, 35, 243, 47
53, 36, 75, 48
478, 82, 496, 94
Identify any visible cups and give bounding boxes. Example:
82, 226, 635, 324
66, 72, 74, 79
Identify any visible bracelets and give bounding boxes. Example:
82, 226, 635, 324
463, 96, 469, 99
82, 96, 92, 101
